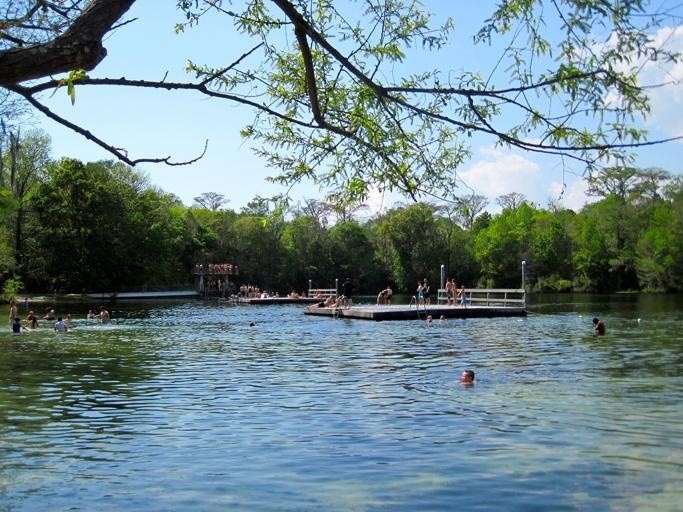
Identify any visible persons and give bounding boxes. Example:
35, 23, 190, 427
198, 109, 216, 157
427, 315, 434, 326
87, 310, 97, 319
43, 306, 55, 321
98, 306, 110, 320
460, 370, 475, 386
287, 277, 356, 309
9, 297, 39, 335
65, 314, 71, 321
196, 263, 279, 298
376, 285, 393, 307
593, 317, 606, 336
445, 278, 468, 309
416, 277, 431, 306
439, 315, 447, 324
54, 317, 67, 333
